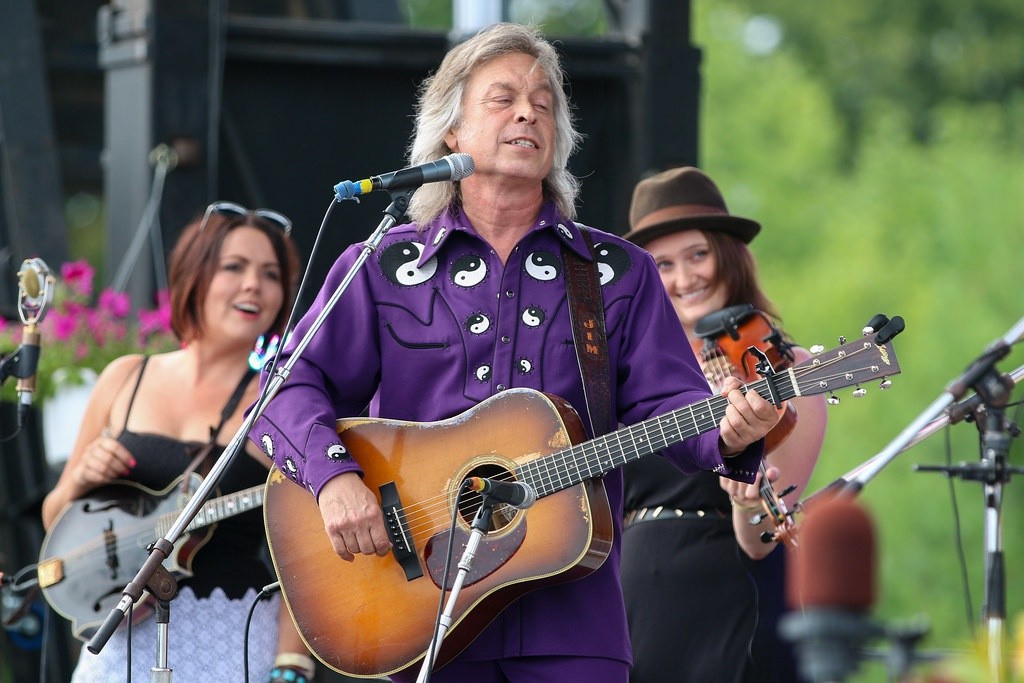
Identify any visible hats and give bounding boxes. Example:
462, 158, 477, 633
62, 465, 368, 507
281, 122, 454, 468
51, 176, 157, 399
621, 167, 761, 249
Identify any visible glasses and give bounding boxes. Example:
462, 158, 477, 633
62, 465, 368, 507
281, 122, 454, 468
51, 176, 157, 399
200, 201, 292, 237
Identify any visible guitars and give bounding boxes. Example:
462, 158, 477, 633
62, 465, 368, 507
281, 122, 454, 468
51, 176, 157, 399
36, 474, 266, 643
262, 311, 905, 683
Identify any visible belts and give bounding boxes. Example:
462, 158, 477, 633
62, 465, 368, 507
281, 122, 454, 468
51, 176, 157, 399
622, 506, 732, 529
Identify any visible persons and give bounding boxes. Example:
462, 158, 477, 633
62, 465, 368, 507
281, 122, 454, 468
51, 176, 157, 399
246, 24, 789, 682
41, 202, 318, 682
448, 2, 650, 226
614, 167, 830, 682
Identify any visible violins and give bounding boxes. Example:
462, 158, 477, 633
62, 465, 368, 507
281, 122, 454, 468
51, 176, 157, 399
688, 303, 808, 552
